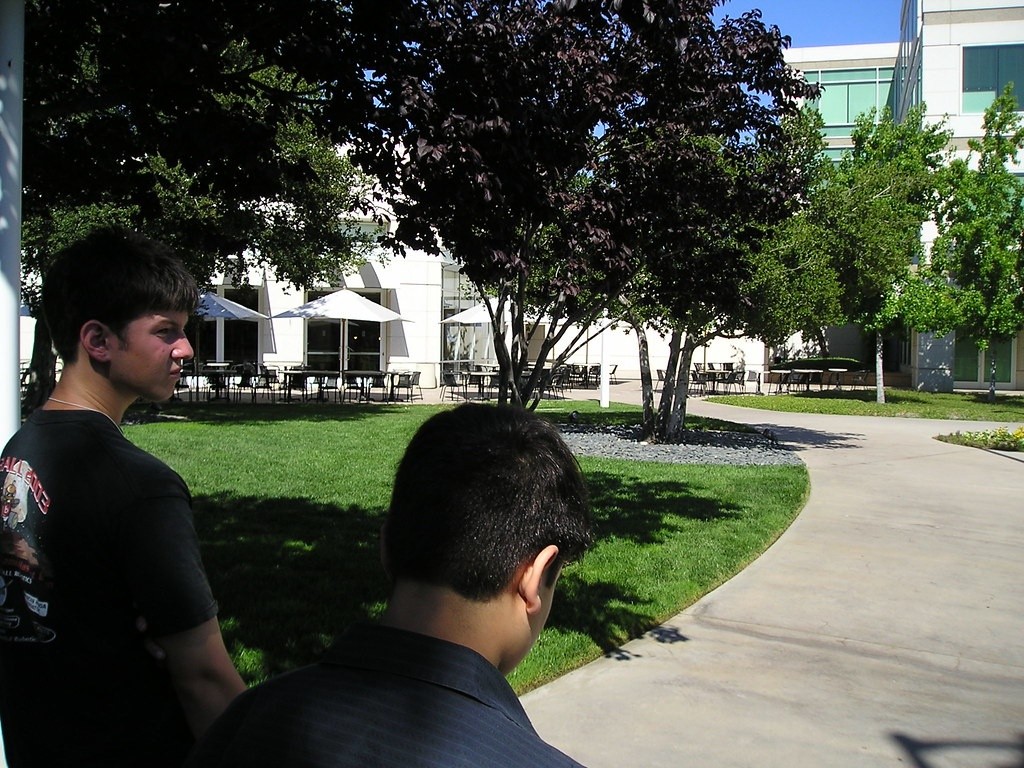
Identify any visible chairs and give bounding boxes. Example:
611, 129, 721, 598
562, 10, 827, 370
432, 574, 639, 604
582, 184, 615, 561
852, 369, 870, 389
173, 362, 424, 403
438, 363, 619, 403
654, 363, 758, 396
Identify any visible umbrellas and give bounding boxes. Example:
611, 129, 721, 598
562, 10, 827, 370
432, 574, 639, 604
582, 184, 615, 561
183, 289, 419, 399
439, 295, 549, 396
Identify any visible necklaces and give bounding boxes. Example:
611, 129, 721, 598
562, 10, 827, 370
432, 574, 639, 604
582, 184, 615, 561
49, 397, 128, 438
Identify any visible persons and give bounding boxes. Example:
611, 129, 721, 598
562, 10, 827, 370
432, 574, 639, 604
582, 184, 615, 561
185, 395, 592, 768
0, 229, 246, 768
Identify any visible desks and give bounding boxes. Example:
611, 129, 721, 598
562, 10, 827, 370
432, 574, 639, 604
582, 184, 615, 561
279, 370, 339, 403
755, 368, 847, 393
202, 369, 237, 403
698, 369, 732, 395
344, 370, 383, 404
462, 371, 499, 401
